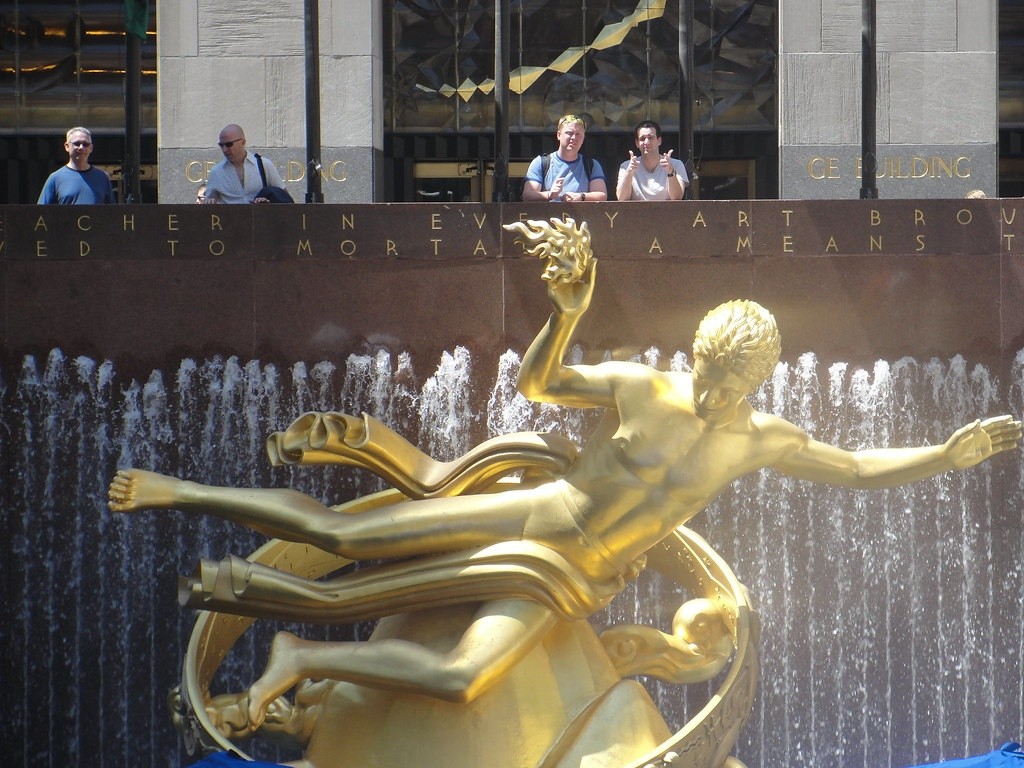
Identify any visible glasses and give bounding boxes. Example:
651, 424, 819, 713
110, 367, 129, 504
217, 138, 242, 148
197, 196, 205, 201
68, 141, 92, 148
560, 114, 586, 130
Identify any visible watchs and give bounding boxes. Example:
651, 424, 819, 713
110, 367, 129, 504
580, 193, 585, 201
667, 169, 675, 177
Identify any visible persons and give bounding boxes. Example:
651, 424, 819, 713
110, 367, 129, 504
965, 190, 986, 198
616, 121, 691, 201
107, 249, 1023, 730
203, 124, 285, 204
37, 127, 116, 205
523, 114, 608, 202
196, 184, 207, 204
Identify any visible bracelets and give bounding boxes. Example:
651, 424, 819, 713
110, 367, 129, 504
548, 193, 553, 200
